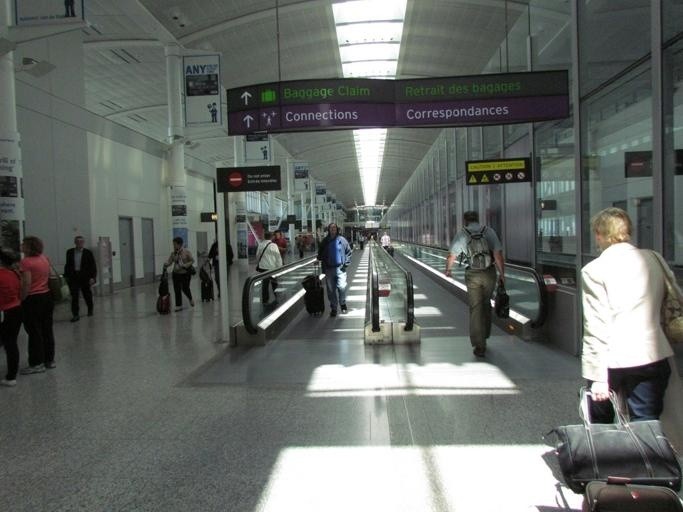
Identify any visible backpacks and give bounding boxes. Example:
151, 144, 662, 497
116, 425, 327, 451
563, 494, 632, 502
200, 261, 212, 280
461, 225, 491, 272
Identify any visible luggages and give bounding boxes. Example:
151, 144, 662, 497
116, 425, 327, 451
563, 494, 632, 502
201, 258, 214, 302
156, 264, 170, 312
579, 385, 683, 512
304, 262, 324, 314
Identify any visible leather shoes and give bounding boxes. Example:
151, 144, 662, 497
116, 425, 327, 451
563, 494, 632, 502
70, 315, 80, 321
473, 345, 485, 356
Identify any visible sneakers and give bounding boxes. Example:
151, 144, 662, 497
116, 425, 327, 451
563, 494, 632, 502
175, 307, 182, 311
45, 361, 57, 368
330, 309, 337, 316
341, 304, 347, 313
2, 379, 17, 386
189, 300, 194, 306
20, 364, 46, 374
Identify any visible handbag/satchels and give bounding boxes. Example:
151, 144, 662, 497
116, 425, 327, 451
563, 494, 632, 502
302, 275, 320, 290
651, 250, 683, 342
188, 264, 196, 275
49, 274, 70, 303
159, 275, 169, 295
495, 279, 509, 318
547, 386, 682, 493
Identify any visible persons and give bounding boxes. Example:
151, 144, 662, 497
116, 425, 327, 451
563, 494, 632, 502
17, 236, 59, 375
0, 248, 24, 386
255, 231, 283, 307
577, 207, 682, 425
312, 222, 354, 318
443, 209, 508, 358
163, 235, 197, 312
205, 234, 233, 297
64, 235, 98, 322
270, 227, 393, 265
